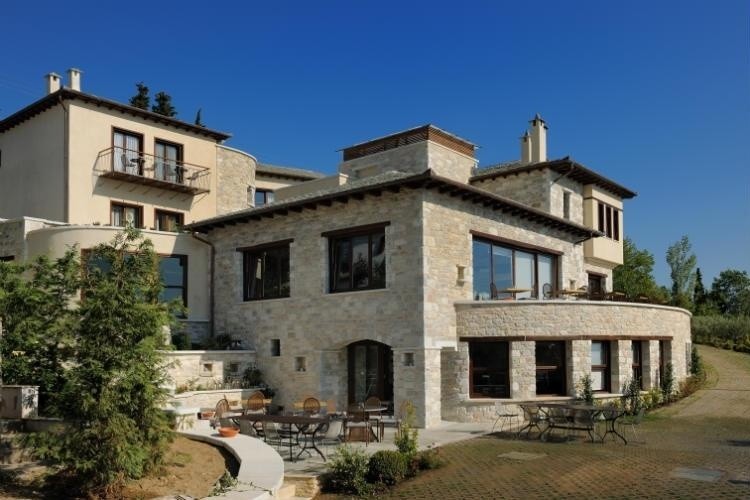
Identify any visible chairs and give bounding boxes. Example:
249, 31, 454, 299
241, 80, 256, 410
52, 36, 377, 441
120, 152, 203, 187
489, 394, 649, 446
213, 392, 409, 464
487, 282, 649, 304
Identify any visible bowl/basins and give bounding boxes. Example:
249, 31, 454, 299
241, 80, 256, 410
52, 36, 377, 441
218, 427, 241, 437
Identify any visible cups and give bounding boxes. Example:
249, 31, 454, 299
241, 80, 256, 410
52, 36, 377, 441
212, 380, 249, 389
